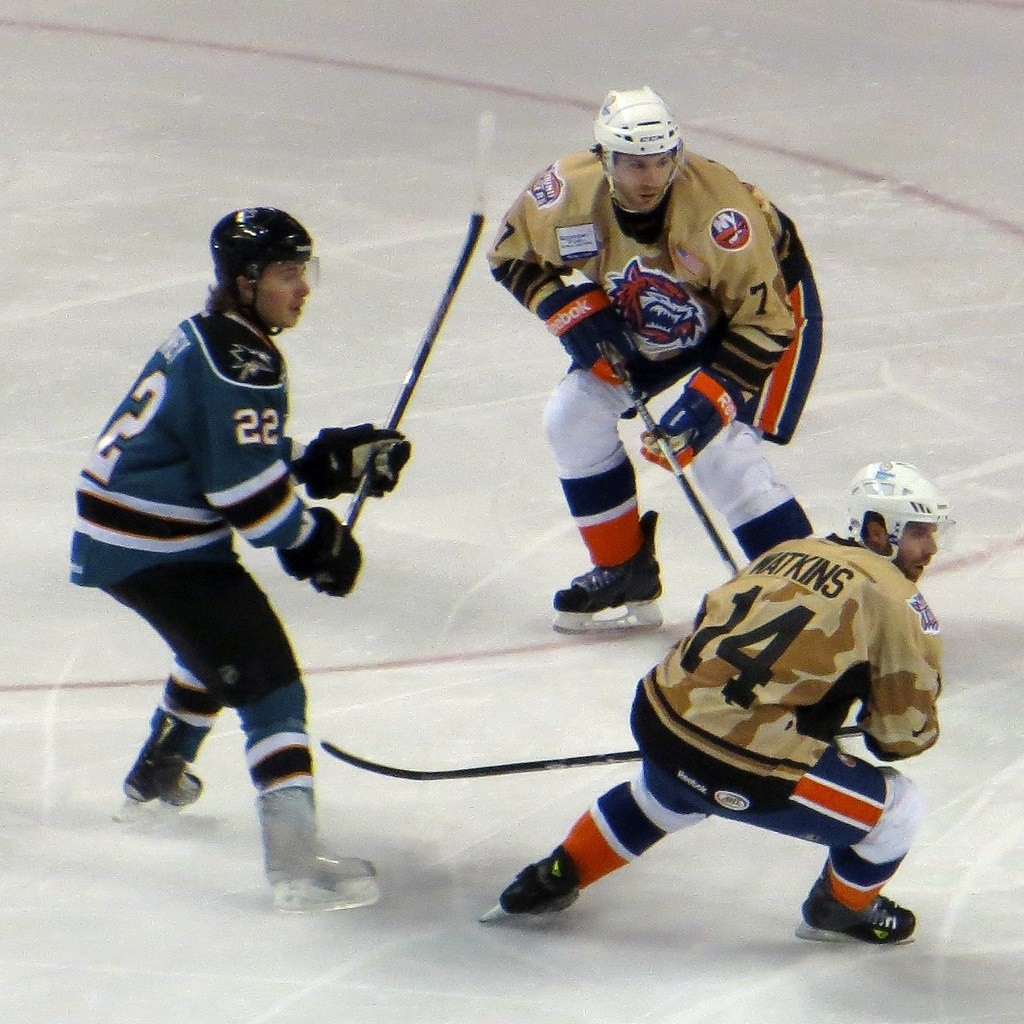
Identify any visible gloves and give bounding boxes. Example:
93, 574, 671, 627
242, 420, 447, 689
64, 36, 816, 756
535, 283, 636, 385
307, 424, 411, 497
276, 507, 360, 597
640, 368, 744, 472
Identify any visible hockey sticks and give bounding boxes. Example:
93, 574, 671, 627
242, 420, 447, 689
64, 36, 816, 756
309, 111, 496, 594
320, 725, 864, 781
595, 334, 740, 576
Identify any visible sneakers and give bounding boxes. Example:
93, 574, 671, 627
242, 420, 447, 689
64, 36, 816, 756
111, 713, 202, 822
553, 510, 663, 632
478, 845, 582, 923
795, 858, 915, 946
255, 791, 380, 913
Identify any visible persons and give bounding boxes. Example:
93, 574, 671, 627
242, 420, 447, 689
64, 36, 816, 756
72, 207, 409, 913
481, 462, 954, 944
490, 90, 826, 635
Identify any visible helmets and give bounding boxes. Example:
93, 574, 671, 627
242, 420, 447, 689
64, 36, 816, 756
594, 85, 679, 175
210, 207, 311, 275
845, 460, 948, 565
